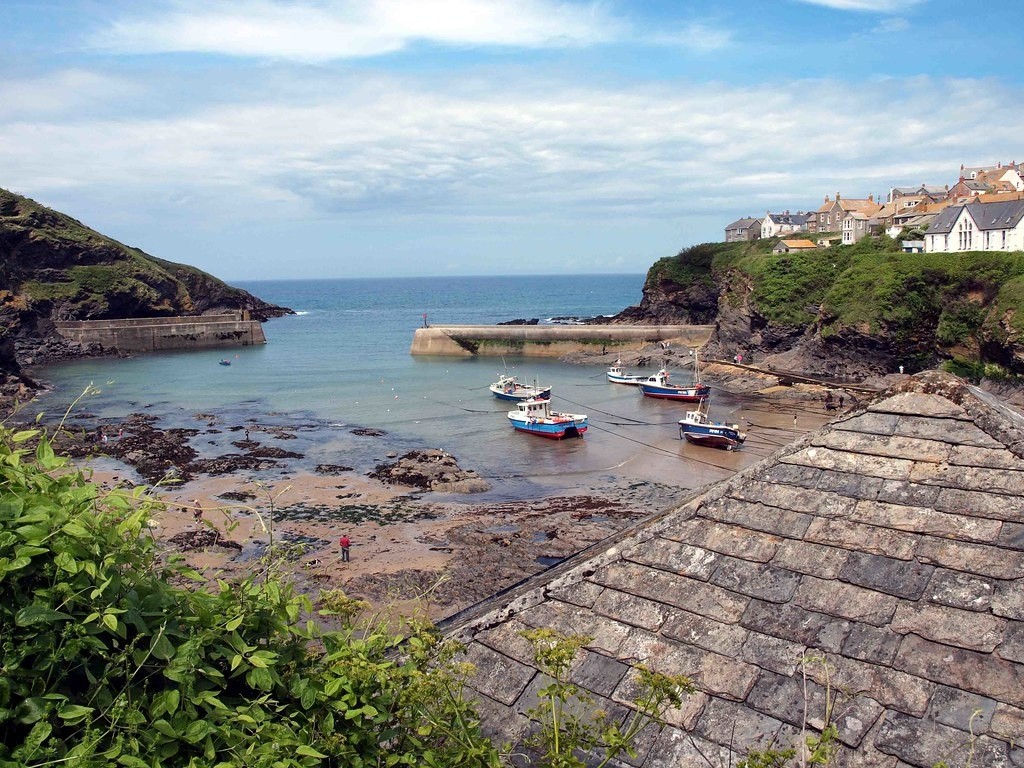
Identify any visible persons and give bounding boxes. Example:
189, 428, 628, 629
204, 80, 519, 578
245, 429, 250, 440
840, 397, 844, 408
794, 414, 798, 424
899, 365, 904, 374
119, 429, 123, 439
96, 425, 108, 444
733, 355, 743, 363
340, 533, 352, 563
193, 499, 204, 524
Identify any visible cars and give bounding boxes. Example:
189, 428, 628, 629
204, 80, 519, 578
775, 378, 792, 386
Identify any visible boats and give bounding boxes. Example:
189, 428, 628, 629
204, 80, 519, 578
489, 356, 553, 404
637, 354, 711, 403
507, 380, 588, 440
607, 359, 670, 387
219, 358, 231, 366
678, 397, 747, 451
